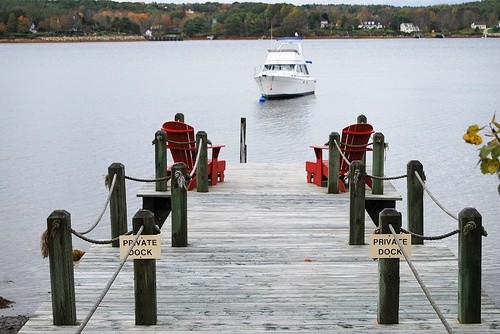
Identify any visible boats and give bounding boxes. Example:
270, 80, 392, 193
252, 36, 316, 98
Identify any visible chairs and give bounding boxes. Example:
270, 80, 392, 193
305, 123, 374, 192
160, 121, 226, 191
